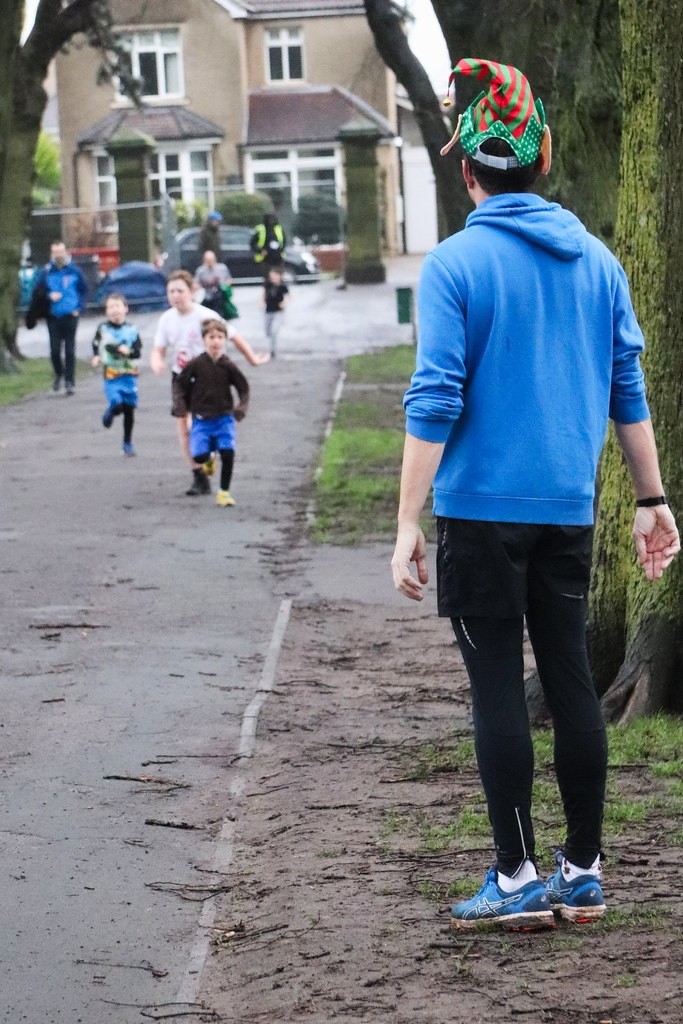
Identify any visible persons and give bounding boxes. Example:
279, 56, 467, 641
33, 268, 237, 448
93, 293, 142, 456
391, 60, 681, 929
193, 250, 233, 315
172, 320, 249, 507
151, 272, 270, 475
198, 212, 223, 262
32, 241, 88, 393
249, 213, 291, 357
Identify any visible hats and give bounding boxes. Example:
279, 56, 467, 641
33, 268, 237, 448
442, 59, 551, 174
209, 213, 222, 221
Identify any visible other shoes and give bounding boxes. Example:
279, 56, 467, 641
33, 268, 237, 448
66, 384, 74, 396
123, 442, 134, 457
53, 374, 61, 389
103, 409, 113, 428
186, 468, 211, 495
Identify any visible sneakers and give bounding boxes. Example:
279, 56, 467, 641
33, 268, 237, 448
450, 866, 555, 932
203, 458, 216, 476
546, 860, 606, 923
216, 490, 236, 507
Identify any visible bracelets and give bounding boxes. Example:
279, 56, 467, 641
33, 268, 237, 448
636, 495, 667, 507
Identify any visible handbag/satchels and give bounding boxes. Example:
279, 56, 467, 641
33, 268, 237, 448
214, 296, 239, 324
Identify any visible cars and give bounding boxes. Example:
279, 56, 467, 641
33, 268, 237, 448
157, 227, 321, 282
